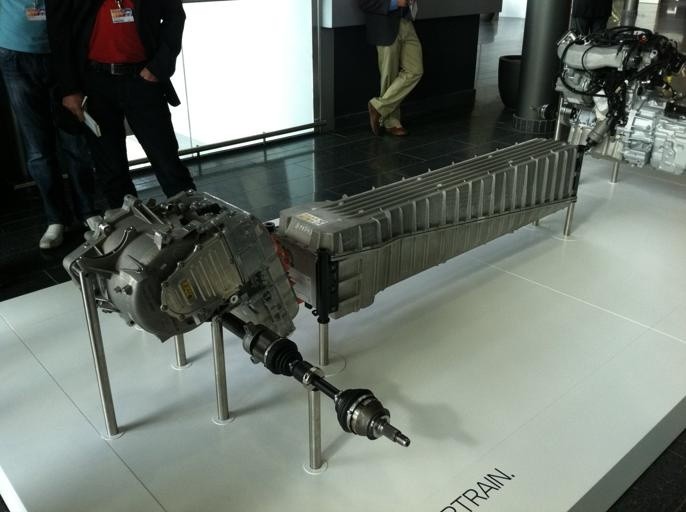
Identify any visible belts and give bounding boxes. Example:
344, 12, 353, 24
87, 57, 145, 76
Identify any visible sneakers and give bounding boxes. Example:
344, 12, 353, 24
37, 220, 69, 249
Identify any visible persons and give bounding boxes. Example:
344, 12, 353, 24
43, 0, 197, 210
1, 0, 100, 251
351, 0, 426, 137
570, 0, 614, 37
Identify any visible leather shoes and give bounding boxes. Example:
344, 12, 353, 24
368, 100, 385, 134
386, 127, 407, 137
